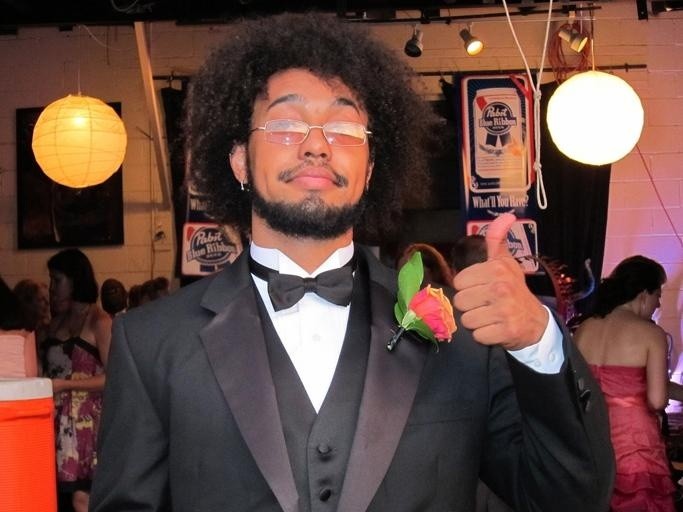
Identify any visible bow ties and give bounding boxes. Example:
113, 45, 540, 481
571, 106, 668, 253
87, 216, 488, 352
267, 265, 354, 313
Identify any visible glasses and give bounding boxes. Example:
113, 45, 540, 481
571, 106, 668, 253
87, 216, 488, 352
250, 118, 374, 148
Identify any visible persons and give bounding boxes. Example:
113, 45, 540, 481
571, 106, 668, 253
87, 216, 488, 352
572, 254, 677, 512
451, 234, 488, 274
90, 10, 615, 512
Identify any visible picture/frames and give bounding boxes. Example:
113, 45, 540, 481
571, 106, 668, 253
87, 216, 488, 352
14, 102, 125, 250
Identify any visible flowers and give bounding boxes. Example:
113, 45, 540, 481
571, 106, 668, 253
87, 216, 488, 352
389, 252, 456, 354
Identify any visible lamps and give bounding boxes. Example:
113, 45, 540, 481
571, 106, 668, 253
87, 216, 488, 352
558, 8, 590, 52
405, 19, 483, 60
28, 1, 128, 192
